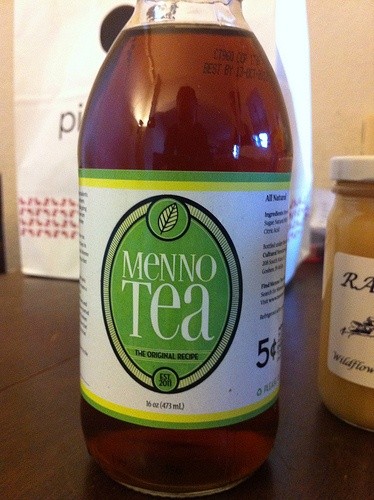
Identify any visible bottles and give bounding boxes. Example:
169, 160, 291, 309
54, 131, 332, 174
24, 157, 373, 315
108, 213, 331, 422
69, 1, 293, 500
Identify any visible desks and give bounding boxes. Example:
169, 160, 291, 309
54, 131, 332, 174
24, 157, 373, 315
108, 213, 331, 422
0, 258, 374, 500
